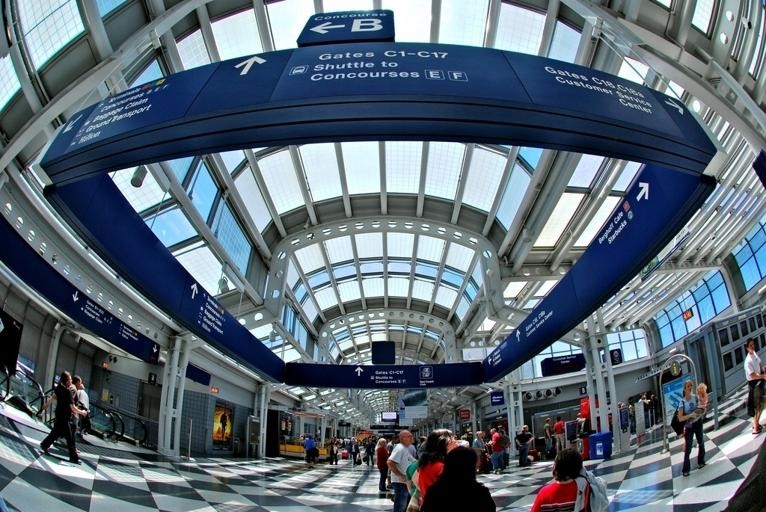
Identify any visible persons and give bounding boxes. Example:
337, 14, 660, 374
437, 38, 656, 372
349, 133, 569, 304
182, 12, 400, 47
330, 436, 376, 466
473, 428, 511, 473
743, 338, 766, 434
531, 448, 610, 512
576, 413, 582, 419
421, 446, 496, 512
678, 380, 705, 476
40, 370, 90, 464
618, 402, 636, 433
688, 383, 708, 422
376, 429, 469, 512
514, 417, 564, 466
642, 394, 660, 428
304, 433, 315, 468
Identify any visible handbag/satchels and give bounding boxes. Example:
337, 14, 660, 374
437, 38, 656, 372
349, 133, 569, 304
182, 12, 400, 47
671, 411, 685, 436
